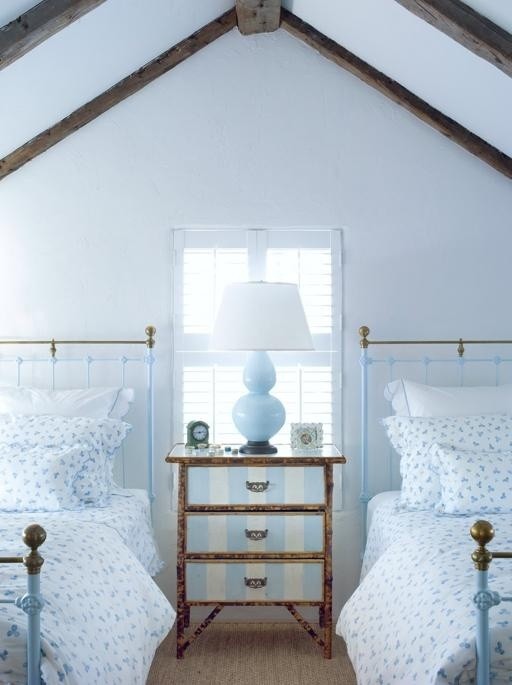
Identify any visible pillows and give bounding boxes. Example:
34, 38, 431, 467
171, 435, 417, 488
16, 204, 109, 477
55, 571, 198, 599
377, 378, 509, 516
2, 386, 136, 513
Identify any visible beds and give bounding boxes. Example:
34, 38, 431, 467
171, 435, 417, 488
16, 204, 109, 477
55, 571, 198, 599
1, 325, 179, 684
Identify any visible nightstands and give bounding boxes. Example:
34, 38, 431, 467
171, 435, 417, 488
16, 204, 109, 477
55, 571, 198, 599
165, 442, 348, 660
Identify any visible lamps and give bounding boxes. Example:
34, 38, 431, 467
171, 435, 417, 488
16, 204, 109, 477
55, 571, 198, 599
209, 280, 316, 455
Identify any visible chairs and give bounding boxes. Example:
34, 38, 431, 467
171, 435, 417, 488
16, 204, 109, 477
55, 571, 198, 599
335, 326, 511, 684
